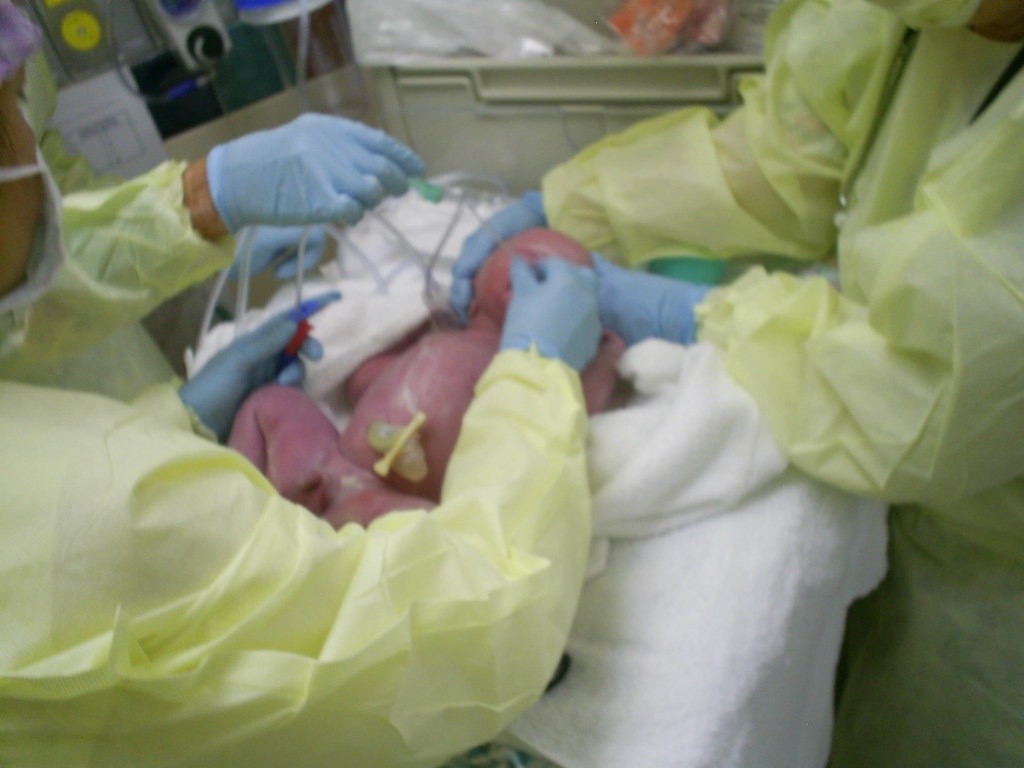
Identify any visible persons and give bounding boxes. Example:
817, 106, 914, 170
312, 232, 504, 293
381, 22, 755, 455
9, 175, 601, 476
0, 0, 604, 768
225, 227, 624, 533
0, 0, 428, 398
450, 0, 1024, 768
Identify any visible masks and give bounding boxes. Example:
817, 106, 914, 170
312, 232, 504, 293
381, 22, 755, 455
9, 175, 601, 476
0, 144, 65, 319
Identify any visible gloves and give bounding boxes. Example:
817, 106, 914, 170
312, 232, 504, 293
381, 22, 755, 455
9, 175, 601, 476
205, 112, 426, 236
592, 252, 713, 348
500, 256, 603, 372
219, 224, 326, 286
449, 191, 546, 329
177, 288, 343, 441
